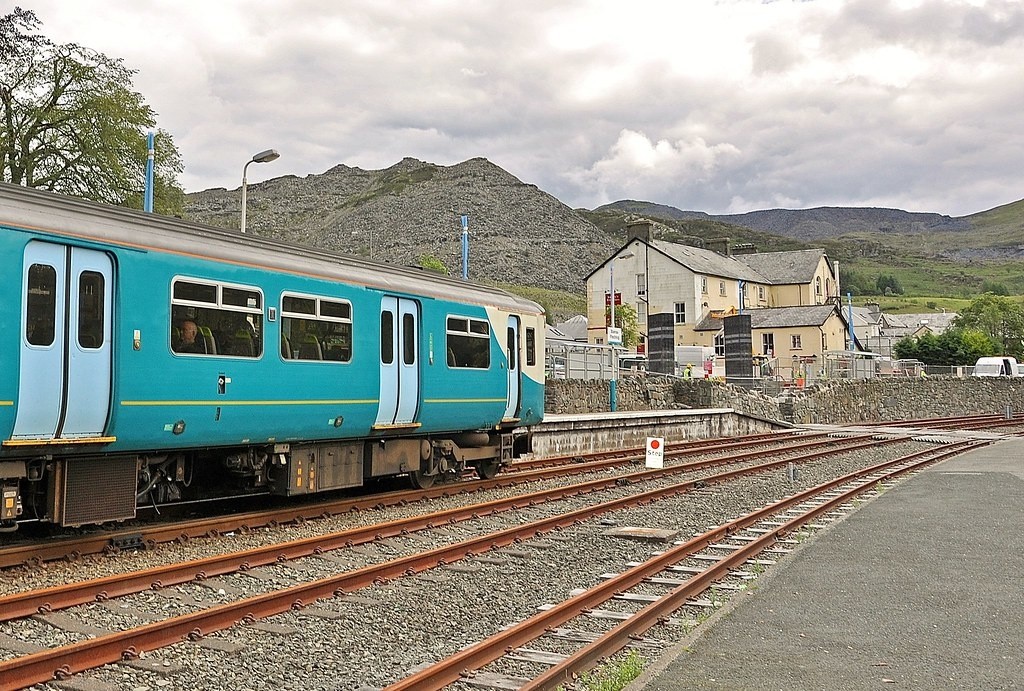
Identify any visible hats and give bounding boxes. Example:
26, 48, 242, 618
921, 368, 924, 370
687, 363, 692, 368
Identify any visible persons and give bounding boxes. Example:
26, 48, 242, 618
172, 321, 206, 355
683, 363, 692, 381
919, 368, 927, 377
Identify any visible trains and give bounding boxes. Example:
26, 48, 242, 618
1, 179, 545, 541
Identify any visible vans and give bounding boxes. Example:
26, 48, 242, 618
972, 356, 1019, 376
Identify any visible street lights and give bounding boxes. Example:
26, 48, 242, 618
240, 148, 281, 234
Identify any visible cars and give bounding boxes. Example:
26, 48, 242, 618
1018, 364, 1024, 377
